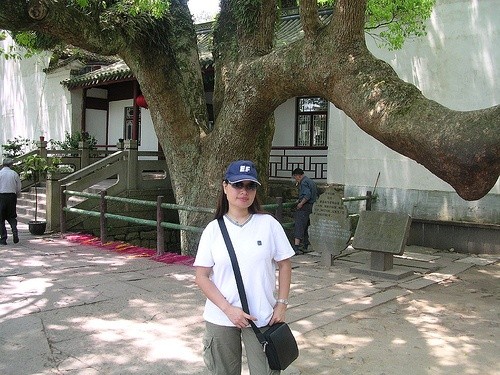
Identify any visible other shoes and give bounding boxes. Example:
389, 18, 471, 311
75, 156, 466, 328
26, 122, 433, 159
0, 239, 6, 245
14, 237, 19, 243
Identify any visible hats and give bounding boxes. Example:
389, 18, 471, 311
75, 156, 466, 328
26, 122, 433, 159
3, 158, 15, 167
223, 158, 261, 186
227, 181, 258, 191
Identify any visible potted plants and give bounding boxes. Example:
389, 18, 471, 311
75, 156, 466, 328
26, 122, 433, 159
22, 155, 48, 235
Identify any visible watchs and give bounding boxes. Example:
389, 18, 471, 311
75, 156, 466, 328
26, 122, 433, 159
276, 299, 289, 305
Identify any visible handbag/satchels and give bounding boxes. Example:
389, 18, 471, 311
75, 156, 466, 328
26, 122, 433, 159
262, 322, 300, 371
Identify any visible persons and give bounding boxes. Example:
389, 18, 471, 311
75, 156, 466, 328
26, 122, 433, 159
292, 168, 316, 250
0, 158, 22, 245
193, 160, 295, 375
